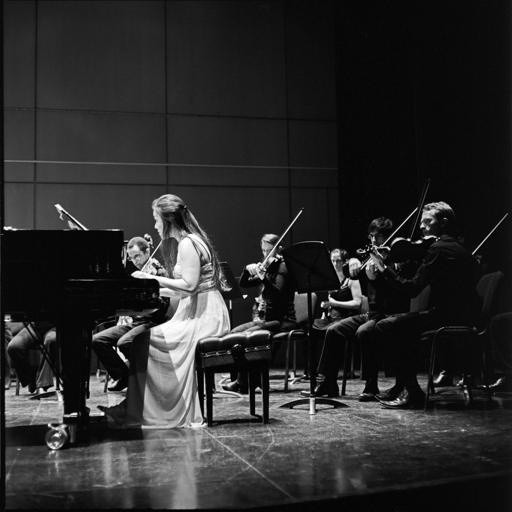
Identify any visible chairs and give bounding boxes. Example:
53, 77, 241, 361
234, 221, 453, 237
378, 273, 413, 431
421, 270, 503, 411
274, 291, 317, 391
341, 296, 368, 396
409, 284, 431, 313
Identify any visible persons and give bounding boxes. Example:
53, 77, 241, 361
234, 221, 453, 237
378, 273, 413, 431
105, 194, 231, 433
476, 302, 512, 392
431, 369, 453, 387
313, 248, 361, 370
300, 216, 408, 401
371, 202, 470, 408
224, 232, 294, 392
7, 318, 57, 399
93, 237, 167, 390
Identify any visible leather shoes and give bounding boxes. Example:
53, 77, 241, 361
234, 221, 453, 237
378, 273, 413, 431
479, 376, 512, 394
433, 374, 454, 388
358, 385, 380, 402
300, 382, 340, 398
374, 385, 404, 402
221, 379, 248, 395
107, 376, 128, 392
378, 386, 427, 411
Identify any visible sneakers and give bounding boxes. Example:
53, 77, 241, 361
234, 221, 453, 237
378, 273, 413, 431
96, 405, 123, 422
107, 417, 142, 432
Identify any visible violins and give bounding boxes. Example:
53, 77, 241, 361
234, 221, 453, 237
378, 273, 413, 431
359, 251, 387, 282
252, 253, 282, 282
366, 235, 436, 272
137, 259, 161, 279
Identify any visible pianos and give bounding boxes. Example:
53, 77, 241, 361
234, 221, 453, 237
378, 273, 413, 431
1, 227, 162, 438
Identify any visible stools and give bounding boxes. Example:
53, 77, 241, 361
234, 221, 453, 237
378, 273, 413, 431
197, 331, 273, 427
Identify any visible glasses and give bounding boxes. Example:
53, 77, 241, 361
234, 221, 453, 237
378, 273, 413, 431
368, 232, 383, 240
126, 253, 145, 262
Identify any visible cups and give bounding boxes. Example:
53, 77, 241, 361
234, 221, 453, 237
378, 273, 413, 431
45, 419, 70, 450
